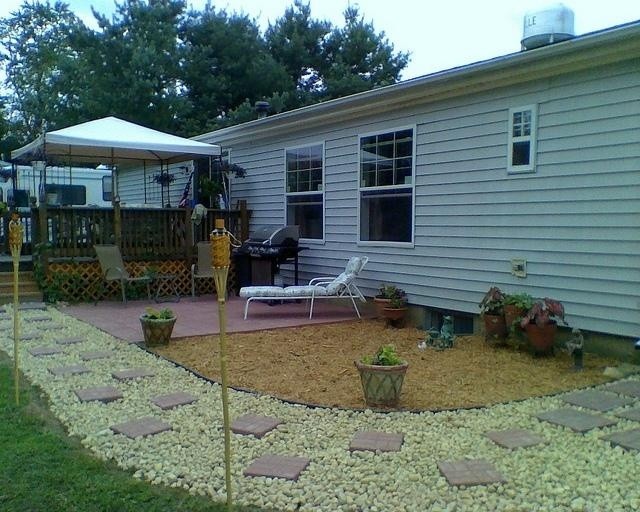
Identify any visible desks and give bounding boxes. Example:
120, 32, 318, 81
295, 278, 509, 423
155, 276, 180, 303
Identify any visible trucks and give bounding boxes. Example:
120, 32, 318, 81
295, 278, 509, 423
1, 164, 116, 253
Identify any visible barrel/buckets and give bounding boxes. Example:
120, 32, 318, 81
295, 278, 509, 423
214, 194, 225, 210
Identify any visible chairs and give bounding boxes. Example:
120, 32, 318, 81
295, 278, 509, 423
239, 255, 370, 320
92, 244, 153, 308
191, 240, 214, 302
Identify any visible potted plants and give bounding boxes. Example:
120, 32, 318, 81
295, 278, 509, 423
479, 286, 564, 350
354, 344, 409, 408
154, 172, 176, 186
373, 283, 408, 328
139, 306, 177, 346
